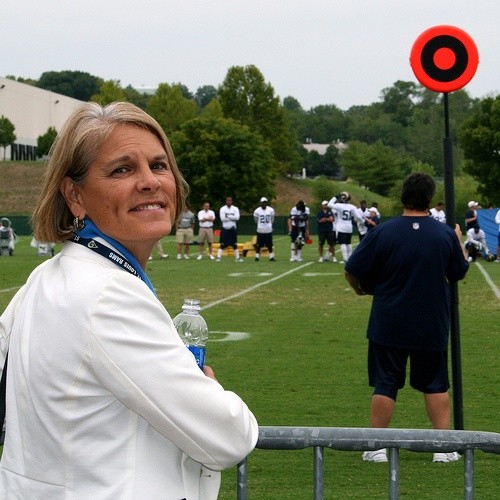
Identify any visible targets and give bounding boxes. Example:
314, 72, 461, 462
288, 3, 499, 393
410, 25, 479, 93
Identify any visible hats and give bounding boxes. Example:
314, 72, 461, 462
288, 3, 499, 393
467, 200, 478, 208
368, 207, 377, 214
260, 197, 268, 202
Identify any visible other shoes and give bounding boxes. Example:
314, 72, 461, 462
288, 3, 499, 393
319, 256, 323, 262
177, 253, 189, 259
160, 253, 168, 259
332, 256, 338, 263
235, 257, 244, 262
210, 254, 215, 259
197, 254, 202, 260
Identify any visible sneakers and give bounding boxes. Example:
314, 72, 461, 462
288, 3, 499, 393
361, 447, 389, 463
432, 451, 461, 462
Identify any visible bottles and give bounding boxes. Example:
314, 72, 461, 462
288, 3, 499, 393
172, 298, 209, 372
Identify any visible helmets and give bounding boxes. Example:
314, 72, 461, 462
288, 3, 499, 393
339, 191, 350, 201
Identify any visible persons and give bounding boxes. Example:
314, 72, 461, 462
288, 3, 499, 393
288, 201, 311, 262
254, 197, 276, 262
215, 196, 243, 262
0, 218, 17, 257
197, 202, 215, 259
430, 202, 446, 223
0, 101, 260, 500
176, 201, 195, 260
464, 200, 500, 262
316, 192, 381, 264
148, 240, 169, 260
342, 173, 468, 461
